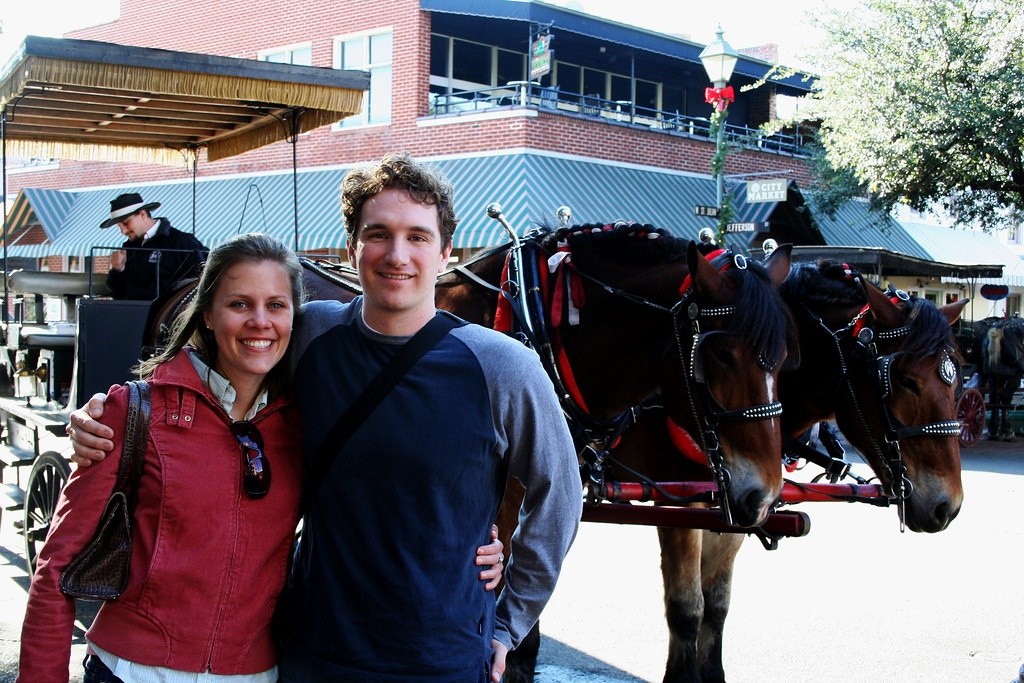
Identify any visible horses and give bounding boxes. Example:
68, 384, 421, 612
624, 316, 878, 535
147, 221, 805, 683
497, 255, 975, 682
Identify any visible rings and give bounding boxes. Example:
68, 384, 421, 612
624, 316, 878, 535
497, 553, 504, 564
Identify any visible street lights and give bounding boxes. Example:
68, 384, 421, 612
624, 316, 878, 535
696, 22, 741, 248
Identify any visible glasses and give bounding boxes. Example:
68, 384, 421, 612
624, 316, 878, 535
230, 420, 271, 499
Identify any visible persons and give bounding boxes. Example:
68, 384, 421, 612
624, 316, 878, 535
65, 154, 582, 683
14, 232, 504, 683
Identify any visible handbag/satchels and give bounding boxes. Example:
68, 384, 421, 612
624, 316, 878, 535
59, 381, 151, 602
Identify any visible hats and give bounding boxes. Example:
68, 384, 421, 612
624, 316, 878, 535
100, 193, 161, 229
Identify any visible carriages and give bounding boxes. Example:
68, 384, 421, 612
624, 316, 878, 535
0, 38, 967, 682
948, 313, 1024, 449
